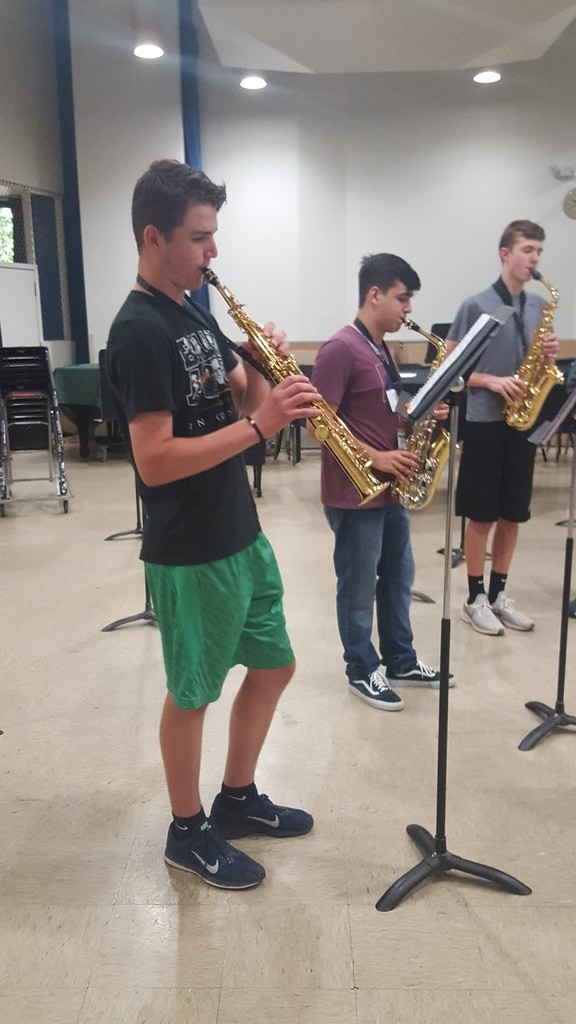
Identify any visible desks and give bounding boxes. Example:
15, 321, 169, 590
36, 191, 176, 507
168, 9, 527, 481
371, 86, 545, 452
52, 363, 102, 456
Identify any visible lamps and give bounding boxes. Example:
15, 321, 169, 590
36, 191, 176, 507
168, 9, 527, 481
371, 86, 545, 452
473, 66, 502, 84
240, 68, 268, 90
133, 0, 164, 60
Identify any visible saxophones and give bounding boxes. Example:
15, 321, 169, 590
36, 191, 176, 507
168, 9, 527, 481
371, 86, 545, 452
198, 268, 389, 506
501, 268, 562, 431
395, 318, 450, 510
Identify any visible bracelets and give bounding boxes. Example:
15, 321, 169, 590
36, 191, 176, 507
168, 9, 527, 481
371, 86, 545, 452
246, 415, 266, 443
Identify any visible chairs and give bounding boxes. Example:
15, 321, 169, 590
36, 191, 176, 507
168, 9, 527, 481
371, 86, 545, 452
1, 346, 72, 514
89, 320, 576, 468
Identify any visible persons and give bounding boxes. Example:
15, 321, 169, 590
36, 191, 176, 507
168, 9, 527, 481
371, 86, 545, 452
105, 158, 326, 889
311, 253, 455, 713
431, 219, 560, 640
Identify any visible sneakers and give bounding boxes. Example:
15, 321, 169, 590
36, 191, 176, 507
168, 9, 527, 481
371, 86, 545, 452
488, 590, 535, 632
210, 793, 312, 840
460, 594, 504, 635
166, 821, 265, 890
386, 660, 454, 688
347, 672, 404, 711
